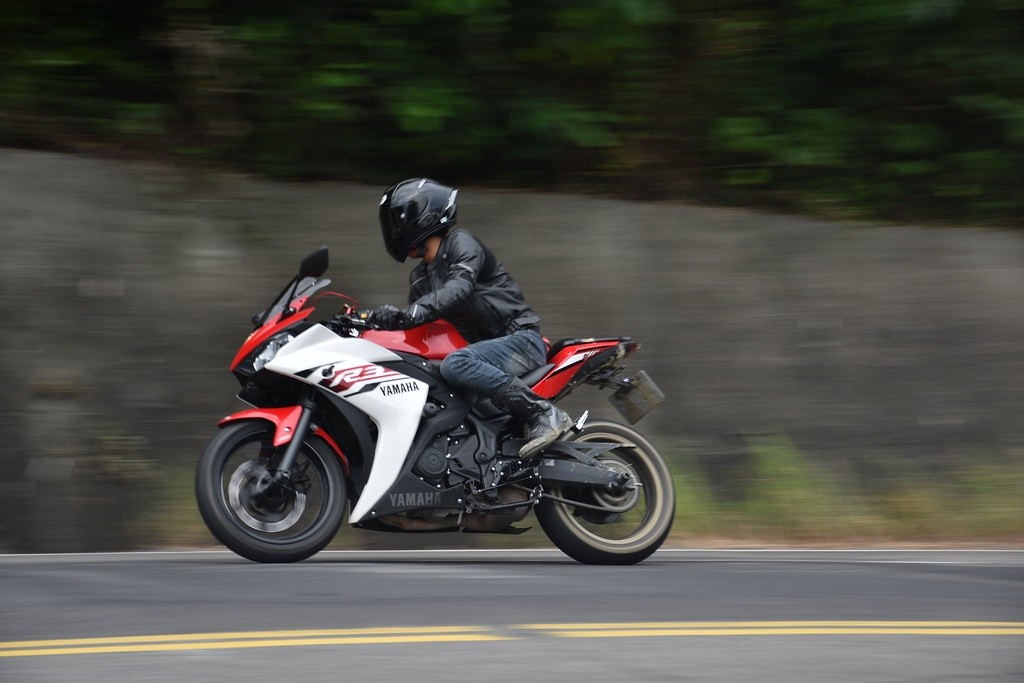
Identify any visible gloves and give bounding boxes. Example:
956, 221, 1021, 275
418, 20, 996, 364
370, 304, 411, 331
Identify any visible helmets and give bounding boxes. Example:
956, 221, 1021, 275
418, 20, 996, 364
379, 177, 459, 263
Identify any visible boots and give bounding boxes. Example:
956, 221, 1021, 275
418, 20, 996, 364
490, 376, 573, 458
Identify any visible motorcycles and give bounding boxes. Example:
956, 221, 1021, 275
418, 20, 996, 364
193, 244, 679, 568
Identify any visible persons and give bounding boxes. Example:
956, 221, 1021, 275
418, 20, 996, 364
332, 177, 573, 460
